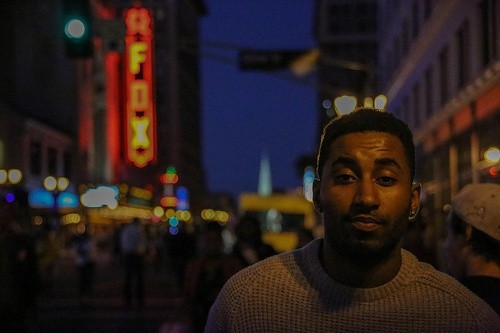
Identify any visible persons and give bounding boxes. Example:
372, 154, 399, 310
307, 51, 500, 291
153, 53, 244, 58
0, 214, 452, 333
203, 106, 500, 333
446, 182, 500, 332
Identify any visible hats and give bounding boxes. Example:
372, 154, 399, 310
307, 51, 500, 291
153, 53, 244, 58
451, 183, 500, 241
